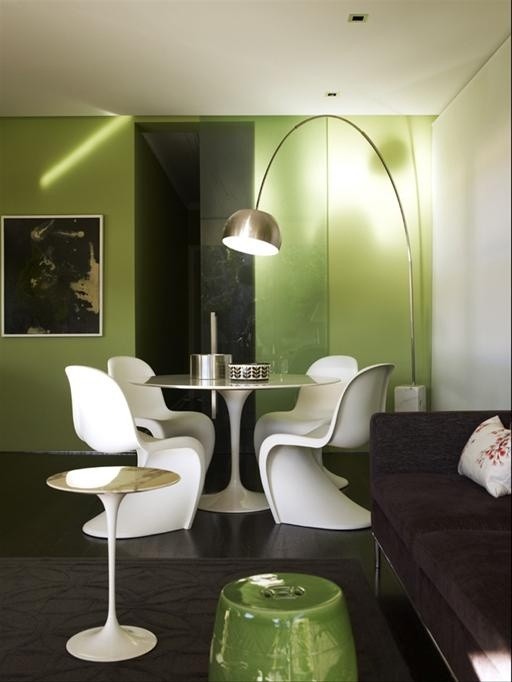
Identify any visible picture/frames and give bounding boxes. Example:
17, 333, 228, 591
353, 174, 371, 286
1, 214, 104, 338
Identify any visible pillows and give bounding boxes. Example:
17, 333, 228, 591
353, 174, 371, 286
456, 415, 511, 499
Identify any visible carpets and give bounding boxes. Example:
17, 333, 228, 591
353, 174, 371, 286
0, 556, 413, 682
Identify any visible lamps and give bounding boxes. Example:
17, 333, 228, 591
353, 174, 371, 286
221, 114, 427, 411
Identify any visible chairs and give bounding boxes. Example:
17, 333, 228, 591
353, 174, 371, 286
258, 363, 395, 531
107, 356, 217, 471
65, 365, 207, 540
253, 355, 359, 490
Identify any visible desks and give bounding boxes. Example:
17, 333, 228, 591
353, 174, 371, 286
127, 373, 342, 514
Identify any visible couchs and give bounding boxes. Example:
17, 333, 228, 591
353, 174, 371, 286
369, 410, 512, 682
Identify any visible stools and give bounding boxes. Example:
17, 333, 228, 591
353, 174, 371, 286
46, 466, 181, 662
207, 572, 361, 682
